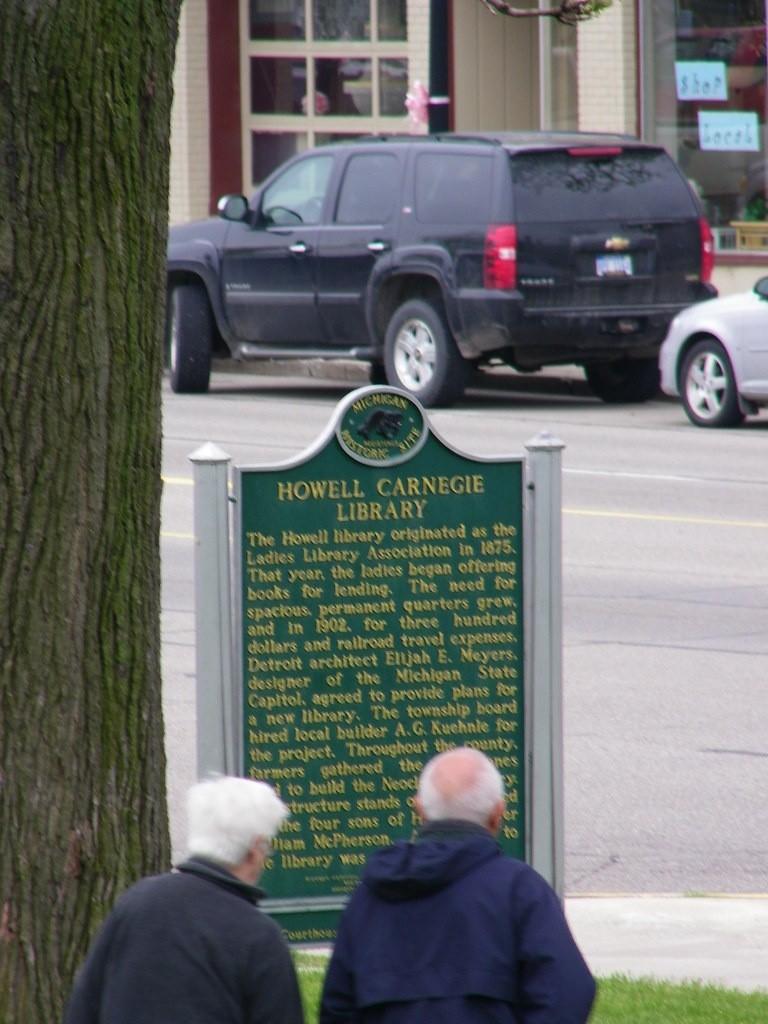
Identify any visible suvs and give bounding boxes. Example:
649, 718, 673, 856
165, 132, 719, 410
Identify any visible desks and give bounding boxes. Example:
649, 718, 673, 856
729, 220, 768, 250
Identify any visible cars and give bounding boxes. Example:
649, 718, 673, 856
659, 275, 768, 430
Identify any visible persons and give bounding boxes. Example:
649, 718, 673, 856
316, 746, 597, 1024
59, 776, 310, 1024
